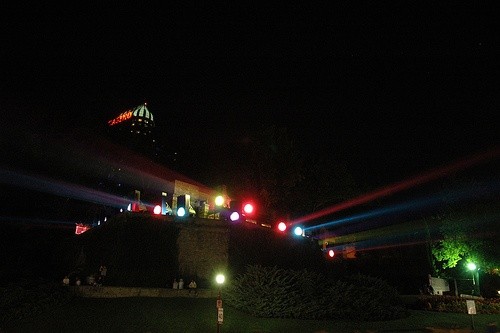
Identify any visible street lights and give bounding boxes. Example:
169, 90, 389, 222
214, 274, 225, 333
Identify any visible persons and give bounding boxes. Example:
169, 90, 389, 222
61, 262, 107, 299
167, 277, 198, 296
